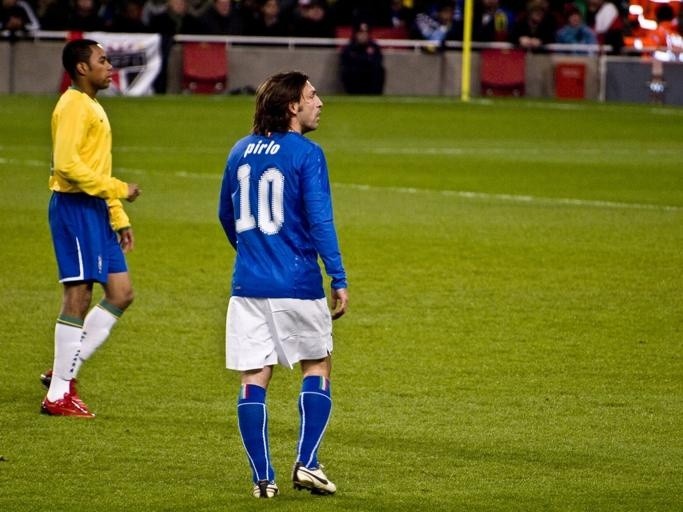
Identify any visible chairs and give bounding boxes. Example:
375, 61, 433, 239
179, 41, 227, 93
479, 47, 526, 96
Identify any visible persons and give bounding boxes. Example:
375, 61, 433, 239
220, 68, 350, 502
40, 35, 141, 417
2, 0, 682, 97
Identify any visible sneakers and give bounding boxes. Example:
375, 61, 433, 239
253, 479, 280, 498
39, 367, 95, 418
293, 462, 337, 495
